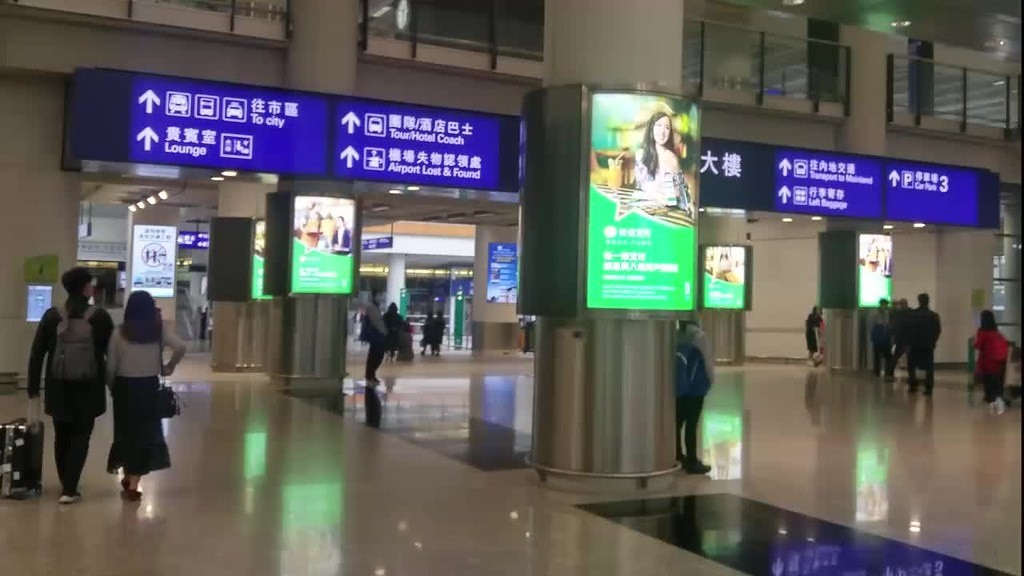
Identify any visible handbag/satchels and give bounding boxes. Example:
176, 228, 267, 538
360, 316, 386, 345
149, 383, 188, 420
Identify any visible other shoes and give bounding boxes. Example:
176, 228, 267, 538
420, 351, 424, 355
985, 403, 995, 414
906, 386, 917, 393
882, 376, 895, 384
390, 353, 395, 358
436, 353, 440, 356
996, 398, 1005, 415
922, 390, 933, 397
57, 488, 81, 503
366, 374, 378, 381
127, 490, 141, 501
686, 460, 711, 474
121, 479, 130, 494
430, 353, 434, 356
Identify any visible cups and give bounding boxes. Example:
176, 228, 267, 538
608, 159, 624, 189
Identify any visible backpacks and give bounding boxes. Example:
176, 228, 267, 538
675, 328, 711, 397
49, 304, 104, 381
980, 326, 1007, 362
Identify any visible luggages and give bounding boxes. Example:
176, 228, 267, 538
0, 398, 44, 501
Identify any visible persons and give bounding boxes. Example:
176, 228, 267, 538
299, 198, 353, 256
676, 313, 711, 476
870, 293, 943, 400
420, 308, 445, 357
382, 301, 414, 361
362, 288, 385, 382
28, 267, 116, 504
631, 111, 696, 221
974, 310, 1007, 415
806, 305, 828, 365
108, 290, 188, 500
